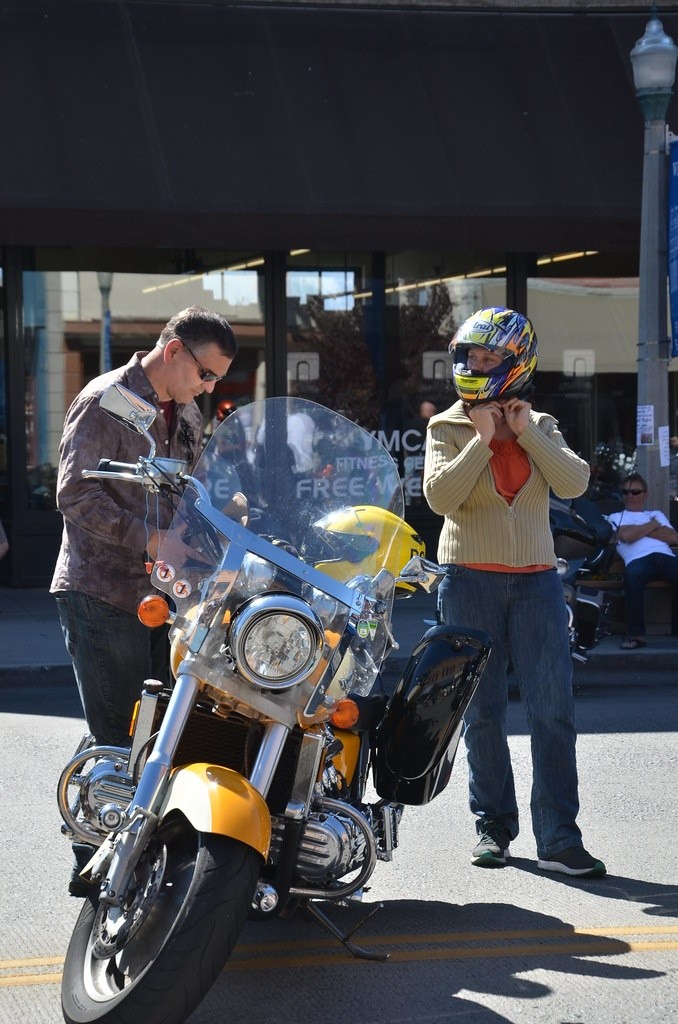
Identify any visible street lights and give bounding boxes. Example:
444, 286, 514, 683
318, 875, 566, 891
629, 18, 678, 524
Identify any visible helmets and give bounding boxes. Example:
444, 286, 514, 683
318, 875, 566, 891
451, 308, 539, 403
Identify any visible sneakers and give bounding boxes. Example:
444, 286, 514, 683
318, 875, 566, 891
538, 846, 607, 874
472, 833, 511, 867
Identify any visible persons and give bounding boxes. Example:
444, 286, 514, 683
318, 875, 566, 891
214, 400, 261, 507
52, 305, 237, 855
420, 306, 609, 877
604, 475, 678, 649
412, 402, 437, 443
669, 436, 677, 449
287, 395, 316, 475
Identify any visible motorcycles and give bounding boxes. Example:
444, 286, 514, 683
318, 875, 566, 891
506, 444, 628, 701
53, 379, 497, 1024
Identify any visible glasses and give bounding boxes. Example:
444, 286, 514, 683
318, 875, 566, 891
622, 488, 643, 496
179, 342, 228, 382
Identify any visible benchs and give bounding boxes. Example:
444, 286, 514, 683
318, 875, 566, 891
575, 545, 678, 637
